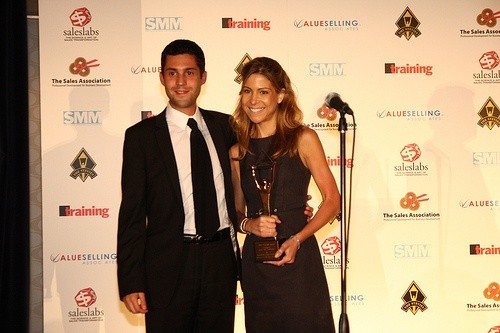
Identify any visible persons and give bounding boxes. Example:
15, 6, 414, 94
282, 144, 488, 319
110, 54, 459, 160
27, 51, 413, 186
228, 57, 341, 333
117, 39, 242, 333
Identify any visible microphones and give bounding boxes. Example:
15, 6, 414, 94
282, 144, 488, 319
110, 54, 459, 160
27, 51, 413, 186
325, 92, 353, 116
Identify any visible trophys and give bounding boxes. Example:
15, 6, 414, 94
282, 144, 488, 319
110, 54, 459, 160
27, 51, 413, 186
250, 149, 282, 262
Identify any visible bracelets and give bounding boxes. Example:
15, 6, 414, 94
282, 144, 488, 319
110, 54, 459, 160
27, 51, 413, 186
290, 235, 300, 249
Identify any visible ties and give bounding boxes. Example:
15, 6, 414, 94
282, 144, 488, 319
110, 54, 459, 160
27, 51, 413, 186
187, 118, 220, 240
